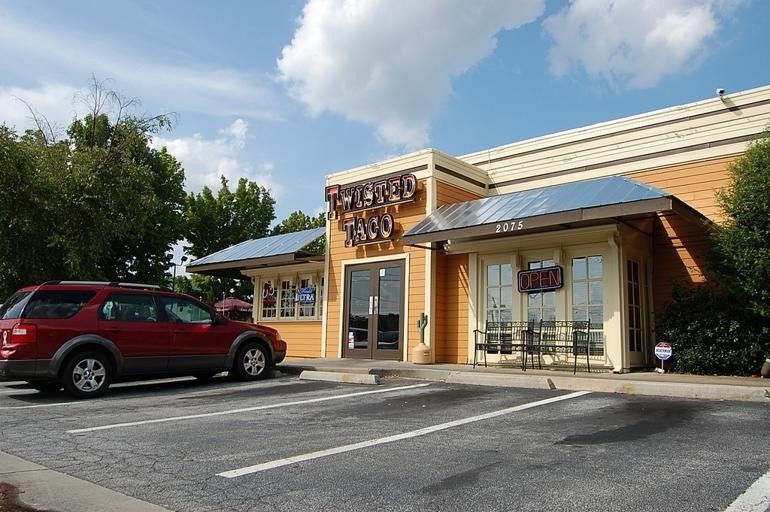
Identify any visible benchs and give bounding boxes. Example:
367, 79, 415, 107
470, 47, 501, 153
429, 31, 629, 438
473, 319, 593, 377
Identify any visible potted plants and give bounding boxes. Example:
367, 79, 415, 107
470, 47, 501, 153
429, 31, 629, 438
411, 310, 432, 364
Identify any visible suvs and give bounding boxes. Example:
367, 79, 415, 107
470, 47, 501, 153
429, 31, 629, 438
348, 327, 400, 350
0, 278, 287, 400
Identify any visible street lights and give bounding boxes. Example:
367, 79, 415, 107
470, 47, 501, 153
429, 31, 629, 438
198, 294, 203, 320
222, 291, 225, 317
167, 254, 188, 313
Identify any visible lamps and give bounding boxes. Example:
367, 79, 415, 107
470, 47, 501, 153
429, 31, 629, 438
717, 88, 727, 100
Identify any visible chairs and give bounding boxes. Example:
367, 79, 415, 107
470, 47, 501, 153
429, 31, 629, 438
111, 304, 153, 320
